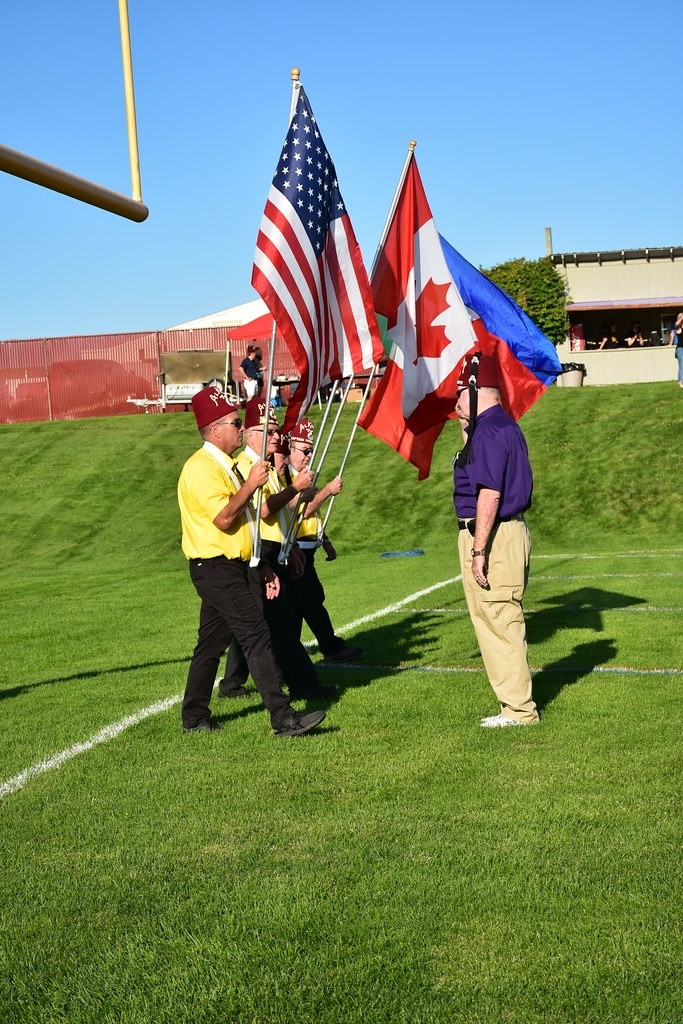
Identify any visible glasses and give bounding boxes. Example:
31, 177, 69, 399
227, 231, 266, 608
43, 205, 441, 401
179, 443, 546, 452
456, 386, 468, 398
251, 428, 280, 436
292, 446, 314, 456
217, 418, 242, 428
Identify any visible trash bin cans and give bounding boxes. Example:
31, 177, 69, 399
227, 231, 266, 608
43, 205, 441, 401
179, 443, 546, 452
562, 363, 584, 387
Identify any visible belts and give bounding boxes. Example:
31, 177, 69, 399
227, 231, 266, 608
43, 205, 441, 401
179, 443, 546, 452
457, 512, 524, 530
262, 540, 281, 550
298, 540, 319, 549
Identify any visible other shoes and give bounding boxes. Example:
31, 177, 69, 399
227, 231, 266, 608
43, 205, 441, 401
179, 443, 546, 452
217, 690, 251, 699
292, 684, 340, 701
324, 645, 364, 664
273, 709, 326, 737
183, 717, 222, 734
479, 716, 540, 729
678, 380, 683, 389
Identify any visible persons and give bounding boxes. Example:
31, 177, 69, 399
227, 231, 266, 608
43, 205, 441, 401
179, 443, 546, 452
595, 323, 624, 349
667, 329, 678, 346
330, 381, 355, 400
626, 320, 645, 348
178, 385, 364, 737
453, 351, 541, 727
675, 313, 683, 388
239, 347, 268, 404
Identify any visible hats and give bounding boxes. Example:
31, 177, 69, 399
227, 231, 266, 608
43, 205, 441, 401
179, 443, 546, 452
290, 418, 315, 445
247, 346, 256, 353
191, 386, 238, 430
456, 352, 500, 467
243, 397, 279, 431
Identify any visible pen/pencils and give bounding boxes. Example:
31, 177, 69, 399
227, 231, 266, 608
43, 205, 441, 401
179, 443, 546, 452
451, 452, 461, 465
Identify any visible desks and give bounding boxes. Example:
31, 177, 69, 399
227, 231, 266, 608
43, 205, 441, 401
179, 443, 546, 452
273, 379, 322, 406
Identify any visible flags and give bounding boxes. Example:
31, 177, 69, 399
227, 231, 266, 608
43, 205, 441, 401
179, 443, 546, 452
356, 153, 563, 481
251, 82, 385, 430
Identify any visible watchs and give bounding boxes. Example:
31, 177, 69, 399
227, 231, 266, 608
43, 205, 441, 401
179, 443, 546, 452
471, 548, 485, 557
